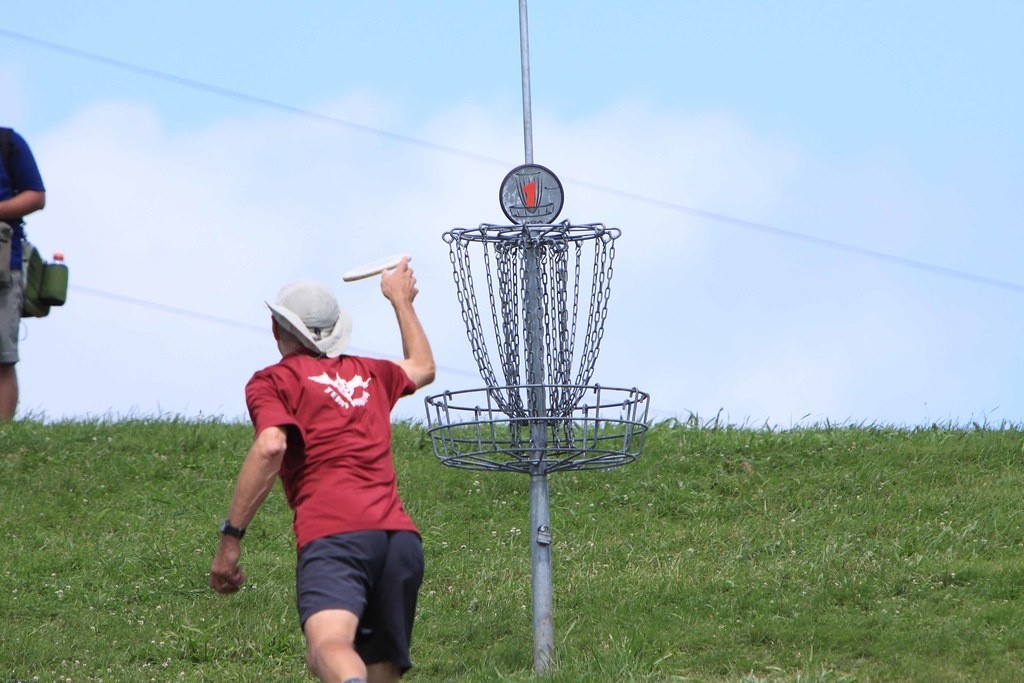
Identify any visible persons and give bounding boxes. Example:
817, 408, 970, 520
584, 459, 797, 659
208, 255, 436, 683
0, 127, 46, 426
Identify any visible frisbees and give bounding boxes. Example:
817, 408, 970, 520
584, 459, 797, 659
343, 256, 411, 282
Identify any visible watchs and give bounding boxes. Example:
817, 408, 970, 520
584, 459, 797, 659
221, 520, 246, 539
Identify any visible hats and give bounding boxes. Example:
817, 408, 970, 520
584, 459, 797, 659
265, 279, 353, 360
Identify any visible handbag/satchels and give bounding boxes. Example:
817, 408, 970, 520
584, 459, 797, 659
19, 235, 68, 319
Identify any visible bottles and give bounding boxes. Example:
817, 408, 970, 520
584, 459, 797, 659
52, 253, 66, 266
